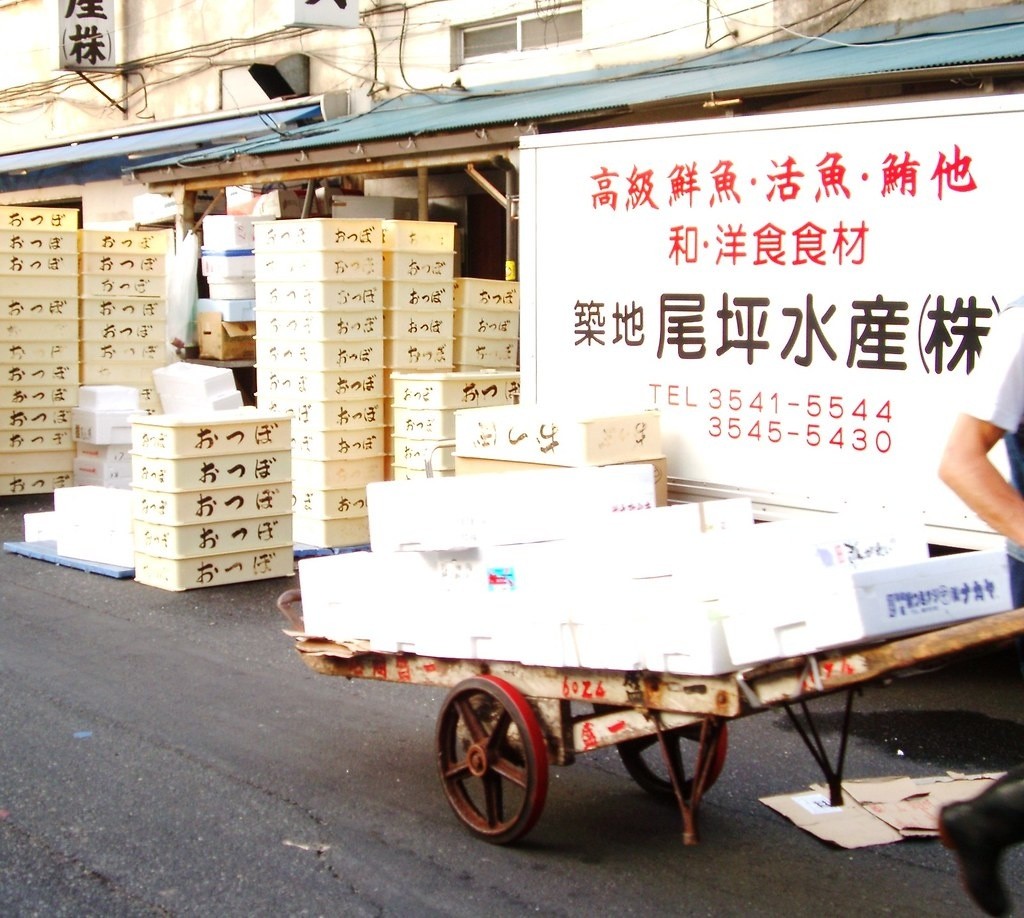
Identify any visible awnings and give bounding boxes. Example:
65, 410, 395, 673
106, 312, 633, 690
122, 0, 1024, 190
0, 104, 321, 192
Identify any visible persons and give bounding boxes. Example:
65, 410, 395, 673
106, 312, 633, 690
935, 300, 1024, 917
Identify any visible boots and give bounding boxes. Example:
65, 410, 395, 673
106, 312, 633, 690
937, 763, 1023, 918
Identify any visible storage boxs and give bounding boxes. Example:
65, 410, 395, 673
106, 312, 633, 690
1, 168, 1022, 679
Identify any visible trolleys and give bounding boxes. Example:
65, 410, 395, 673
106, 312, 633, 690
270, 585, 1024, 845
